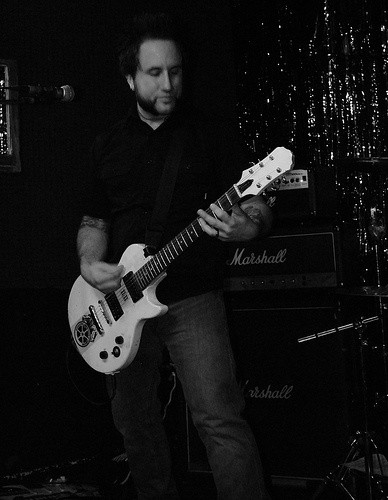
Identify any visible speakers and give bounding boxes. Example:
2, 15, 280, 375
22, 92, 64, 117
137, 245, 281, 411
183, 304, 357, 473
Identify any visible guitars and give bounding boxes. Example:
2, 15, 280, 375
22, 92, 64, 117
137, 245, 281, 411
67, 145, 295, 377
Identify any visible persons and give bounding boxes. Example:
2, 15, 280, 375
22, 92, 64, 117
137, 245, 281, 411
76, 31, 276, 500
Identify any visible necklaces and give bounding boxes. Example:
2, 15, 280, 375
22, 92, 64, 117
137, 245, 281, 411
137, 109, 166, 121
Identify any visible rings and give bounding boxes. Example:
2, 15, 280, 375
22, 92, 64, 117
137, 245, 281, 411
215, 230, 219, 237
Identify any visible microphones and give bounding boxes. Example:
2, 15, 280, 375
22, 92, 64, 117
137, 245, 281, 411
24, 84, 74, 101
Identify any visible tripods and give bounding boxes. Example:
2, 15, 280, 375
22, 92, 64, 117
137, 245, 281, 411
313, 320, 388, 499
